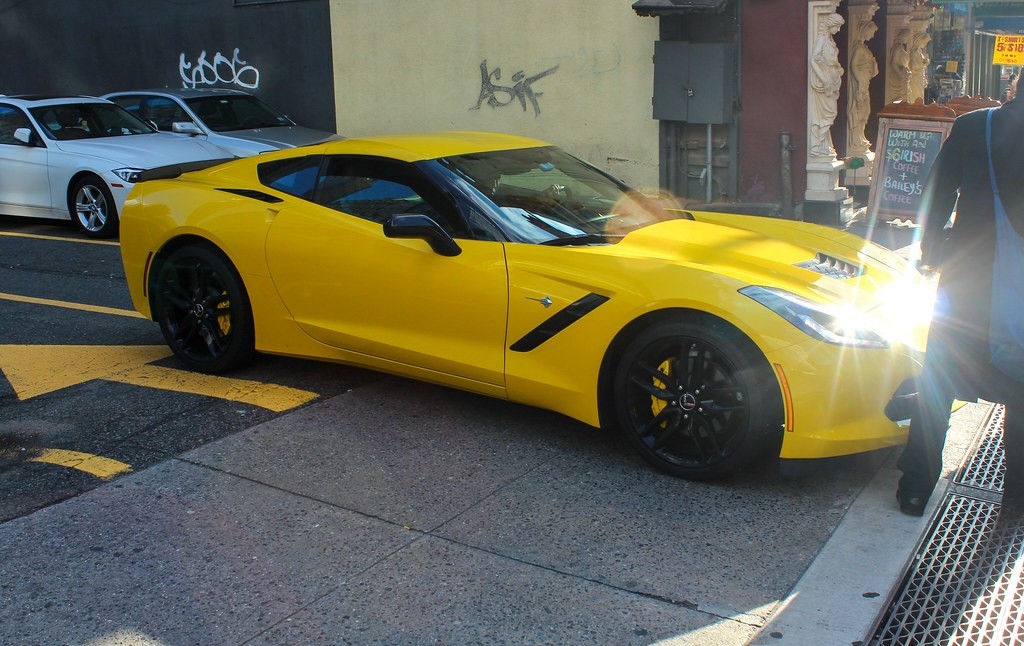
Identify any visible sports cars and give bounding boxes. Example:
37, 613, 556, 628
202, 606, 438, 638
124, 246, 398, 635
118, 131, 948, 481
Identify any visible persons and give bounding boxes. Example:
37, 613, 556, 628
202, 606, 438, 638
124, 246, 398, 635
848, 20, 880, 149
477, 173, 560, 203
897, 65, 1024, 518
811, 14, 846, 161
889, 28, 913, 104
909, 32, 931, 104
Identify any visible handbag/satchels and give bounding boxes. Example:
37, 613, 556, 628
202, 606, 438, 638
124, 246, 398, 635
986, 108, 1024, 383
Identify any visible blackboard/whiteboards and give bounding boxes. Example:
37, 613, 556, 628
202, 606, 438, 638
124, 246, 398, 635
872, 122, 949, 217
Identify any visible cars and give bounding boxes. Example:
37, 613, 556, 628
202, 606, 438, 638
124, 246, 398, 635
101, 85, 347, 195
0, 92, 244, 239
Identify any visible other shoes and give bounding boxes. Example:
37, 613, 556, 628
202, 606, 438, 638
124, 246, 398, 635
1004, 485, 1024, 511
896, 490, 929, 517
